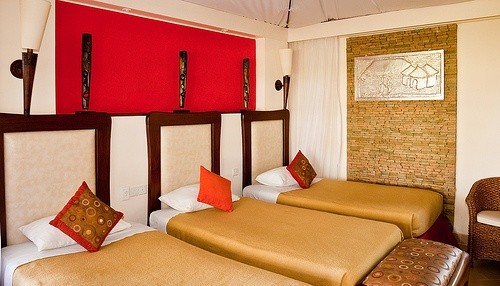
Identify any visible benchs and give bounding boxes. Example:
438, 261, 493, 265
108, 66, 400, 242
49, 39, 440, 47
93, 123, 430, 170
362, 238, 472, 286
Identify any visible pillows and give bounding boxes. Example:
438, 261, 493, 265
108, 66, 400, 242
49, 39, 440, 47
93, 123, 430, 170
157, 184, 240, 212
49, 180, 125, 252
197, 164, 233, 213
18, 213, 131, 254
254, 165, 296, 187
287, 150, 317, 189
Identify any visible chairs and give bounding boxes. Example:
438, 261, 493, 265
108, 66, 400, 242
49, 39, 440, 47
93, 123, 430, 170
465, 177, 500, 266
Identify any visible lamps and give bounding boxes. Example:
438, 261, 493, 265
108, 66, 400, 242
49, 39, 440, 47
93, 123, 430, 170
275, 48, 293, 110
10, 0, 51, 116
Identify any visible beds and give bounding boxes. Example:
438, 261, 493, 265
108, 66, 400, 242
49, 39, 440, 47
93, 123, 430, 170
241, 111, 452, 242
147, 111, 404, 286
0, 111, 314, 286
